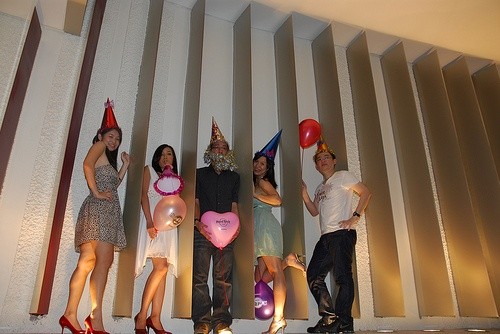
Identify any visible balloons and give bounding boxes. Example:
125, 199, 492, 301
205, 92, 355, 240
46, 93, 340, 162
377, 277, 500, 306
200, 211, 240, 249
255, 280, 275, 321
298, 119, 322, 149
153, 195, 187, 231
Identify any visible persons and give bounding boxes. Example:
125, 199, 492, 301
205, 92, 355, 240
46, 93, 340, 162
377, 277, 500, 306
191, 117, 240, 334
58, 102, 131, 334
252, 129, 307, 334
134, 144, 179, 334
301, 137, 371, 334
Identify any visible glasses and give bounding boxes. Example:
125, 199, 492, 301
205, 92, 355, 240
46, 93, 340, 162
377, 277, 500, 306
315, 156, 332, 162
211, 147, 228, 152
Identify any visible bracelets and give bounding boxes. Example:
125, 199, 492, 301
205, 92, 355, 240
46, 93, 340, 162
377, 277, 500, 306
194, 217, 199, 226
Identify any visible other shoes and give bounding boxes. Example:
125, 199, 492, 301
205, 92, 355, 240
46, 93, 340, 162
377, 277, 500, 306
320, 320, 353, 334
195, 324, 209, 334
215, 326, 233, 334
307, 318, 336, 334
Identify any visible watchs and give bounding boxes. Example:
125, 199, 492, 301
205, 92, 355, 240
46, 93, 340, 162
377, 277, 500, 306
353, 211, 361, 217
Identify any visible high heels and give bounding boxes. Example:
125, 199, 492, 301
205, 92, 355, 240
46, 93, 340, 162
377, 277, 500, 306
285, 252, 307, 271
262, 317, 287, 334
59, 315, 85, 334
84, 314, 109, 334
146, 316, 172, 334
134, 313, 146, 334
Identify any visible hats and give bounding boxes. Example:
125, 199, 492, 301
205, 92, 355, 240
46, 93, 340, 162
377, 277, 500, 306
313, 134, 335, 154
100, 98, 119, 129
210, 116, 226, 143
259, 129, 283, 160
